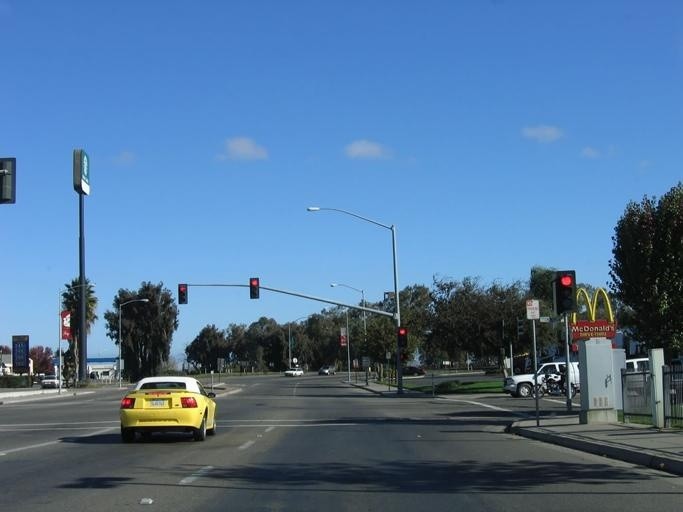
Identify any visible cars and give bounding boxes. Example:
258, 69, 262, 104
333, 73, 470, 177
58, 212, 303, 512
401, 365, 427, 378
119, 375, 217, 443
318, 365, 337, 376
504, 360, 579, 397
41, 375, 63, 389
625, 358, 652, 394
285, 367, 304, 377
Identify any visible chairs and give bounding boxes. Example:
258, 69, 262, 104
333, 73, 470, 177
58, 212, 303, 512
145, 383, 156, 389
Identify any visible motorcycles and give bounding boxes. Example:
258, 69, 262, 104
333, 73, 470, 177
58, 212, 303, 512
531, 371, 576, 399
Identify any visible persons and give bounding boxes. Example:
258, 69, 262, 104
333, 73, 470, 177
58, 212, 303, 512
556, 364, 568, 390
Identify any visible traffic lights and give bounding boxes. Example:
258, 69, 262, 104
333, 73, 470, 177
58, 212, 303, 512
398, 326, 408, 349
556, 270, 577, 312
250, 278, 260, 299
178, 284, 187, 304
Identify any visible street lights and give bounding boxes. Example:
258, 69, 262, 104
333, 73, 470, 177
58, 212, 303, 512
288, 315, 313, 368
307, 205, 402, 393
118, 298, 149, 393
57, 283, 95, 392
